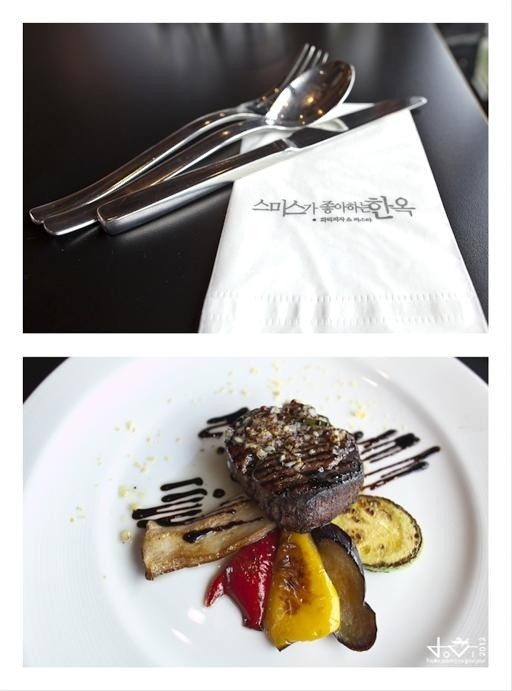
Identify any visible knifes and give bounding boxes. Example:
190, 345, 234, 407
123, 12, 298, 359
95, 93, 430, 234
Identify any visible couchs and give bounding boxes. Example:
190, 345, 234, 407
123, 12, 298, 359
24, 21, 487, 332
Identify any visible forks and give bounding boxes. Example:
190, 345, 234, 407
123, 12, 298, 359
28, 41, 335, 225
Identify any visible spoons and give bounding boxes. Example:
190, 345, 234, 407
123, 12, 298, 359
44, 58, 358, 242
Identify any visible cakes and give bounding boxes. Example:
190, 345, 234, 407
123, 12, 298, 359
223, 401, 364, 534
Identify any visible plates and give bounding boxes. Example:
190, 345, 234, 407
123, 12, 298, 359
25, 359, 488, 670
